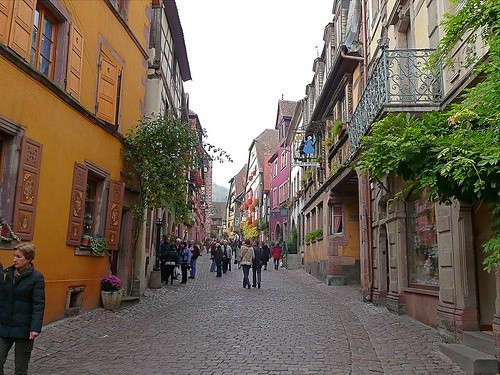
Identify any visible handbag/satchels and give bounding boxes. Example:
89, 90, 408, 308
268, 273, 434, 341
258, 260, 263, 266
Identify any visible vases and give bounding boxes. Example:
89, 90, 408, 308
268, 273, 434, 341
102, 289, 122, 310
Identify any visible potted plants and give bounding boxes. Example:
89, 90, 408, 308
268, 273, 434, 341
82, 233, 112, 256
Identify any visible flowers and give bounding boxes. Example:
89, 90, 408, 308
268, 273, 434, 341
101, 274, 122, 291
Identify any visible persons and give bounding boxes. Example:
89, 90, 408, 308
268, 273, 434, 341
272, 243, 282, 269
0, 242, 47, 375
158, 233, 200, 285
205, 236, 271, 277
249, 240, 265, 289
238, 239, 254, 289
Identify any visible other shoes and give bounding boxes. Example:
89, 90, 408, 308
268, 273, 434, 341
252, 285, 256, 287
248, 284, 251, 289
243, 283, 246, 288
258, 283, 260, 288
